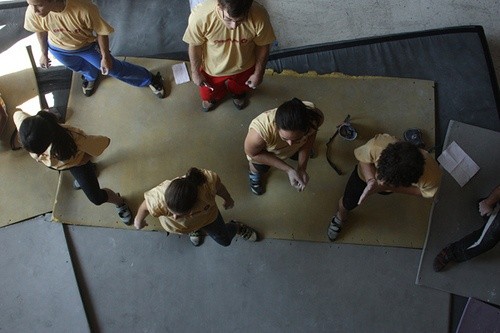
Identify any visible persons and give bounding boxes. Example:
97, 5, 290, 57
433, 184, 500, 272
327, 132, 443, 242
23, 0, 166, 98
244, 97, 324, 195
182, 0, 276, 112
134, 167, 258, 247
13, 108, 133, 226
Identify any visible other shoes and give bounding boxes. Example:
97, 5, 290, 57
116, 199, 133, 227
248, 171, 266, 196
189, 230, 202, 246
229, 219, 258, 243
82, 76, 98, 97
202, 100, 216, 111
72, 162, 97, 191
231, 95, 248, 111
148, 72, 166, 99
432, 247, 452, 273
326, 215, 343, 242
